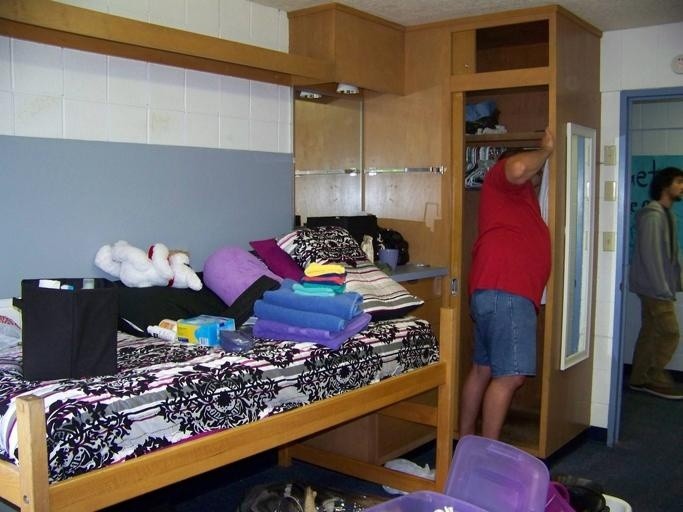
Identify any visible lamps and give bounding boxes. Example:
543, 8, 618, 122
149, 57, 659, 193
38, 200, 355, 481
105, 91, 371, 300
337, 83, 360, 95
298, 91, 323, 100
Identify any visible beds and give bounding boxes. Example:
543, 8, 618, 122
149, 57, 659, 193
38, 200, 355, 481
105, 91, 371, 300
0, 305, 462, 512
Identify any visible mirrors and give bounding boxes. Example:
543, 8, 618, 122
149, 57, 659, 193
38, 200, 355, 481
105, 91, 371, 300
559, 122, 597, 372
291, 87, 365, 233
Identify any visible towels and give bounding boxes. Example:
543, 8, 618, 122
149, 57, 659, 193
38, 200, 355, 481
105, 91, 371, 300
252, 262, 371, 350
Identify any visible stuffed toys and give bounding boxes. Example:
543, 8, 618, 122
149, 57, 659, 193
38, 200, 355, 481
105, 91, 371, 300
94, 239, 203, 291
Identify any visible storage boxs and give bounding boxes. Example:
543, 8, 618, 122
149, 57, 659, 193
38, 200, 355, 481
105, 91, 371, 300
360, 434, 633, 512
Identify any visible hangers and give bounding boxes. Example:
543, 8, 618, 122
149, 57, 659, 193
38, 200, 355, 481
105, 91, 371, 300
462, 143, 506, 189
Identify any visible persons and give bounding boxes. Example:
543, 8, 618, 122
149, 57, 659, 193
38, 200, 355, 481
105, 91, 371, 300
459, 126, 555, 442
628, 167, 683, 400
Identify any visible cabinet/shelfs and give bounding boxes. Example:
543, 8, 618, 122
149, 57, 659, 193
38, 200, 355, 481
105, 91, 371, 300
298, 263, 448, 467
363, 4, 604, 459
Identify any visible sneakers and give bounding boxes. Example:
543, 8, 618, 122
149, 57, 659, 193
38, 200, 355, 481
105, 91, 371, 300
631, 383, 644, 391
643, 382, 683, 399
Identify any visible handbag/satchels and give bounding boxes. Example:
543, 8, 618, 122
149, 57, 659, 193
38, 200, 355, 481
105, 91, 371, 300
22, 279, 119, 381
374, 227, 409, 265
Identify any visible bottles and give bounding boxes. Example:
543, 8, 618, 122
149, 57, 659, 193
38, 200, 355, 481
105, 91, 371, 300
147, 318, 177, 341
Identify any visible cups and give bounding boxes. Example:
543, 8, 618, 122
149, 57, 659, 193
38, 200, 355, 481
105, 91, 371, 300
378, 249, 399, 271
